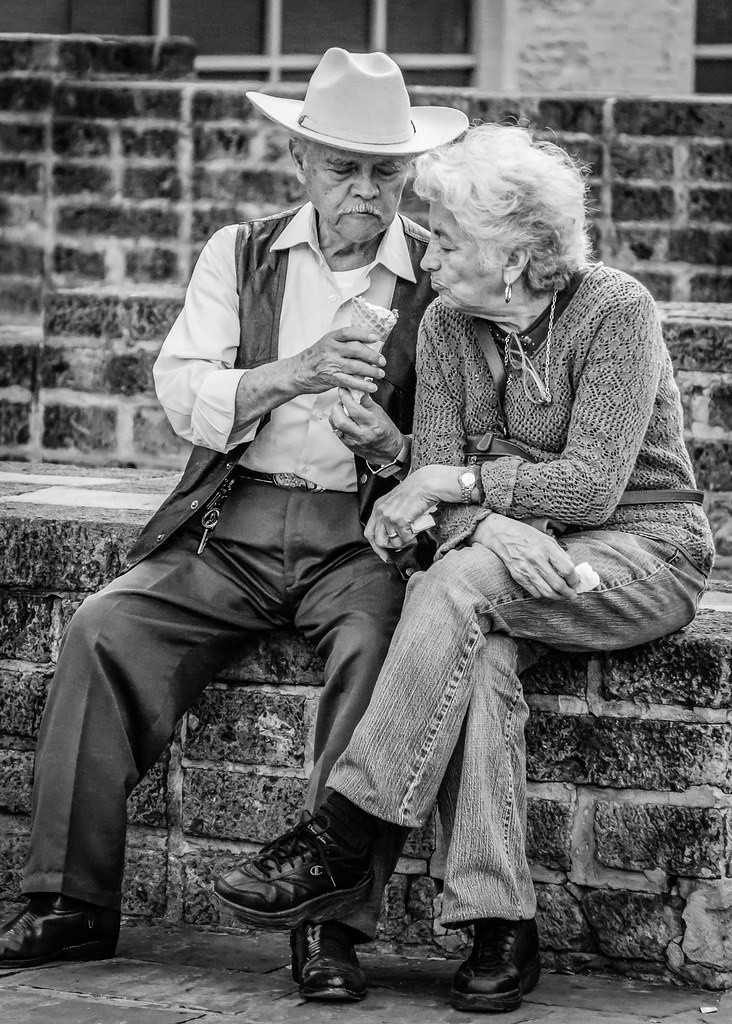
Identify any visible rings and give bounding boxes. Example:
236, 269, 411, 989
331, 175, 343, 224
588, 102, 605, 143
388, 532, 398, 538
333, 429, 344, 438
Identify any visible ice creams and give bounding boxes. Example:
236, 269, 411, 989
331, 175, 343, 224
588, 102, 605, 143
348, 296, 399, 344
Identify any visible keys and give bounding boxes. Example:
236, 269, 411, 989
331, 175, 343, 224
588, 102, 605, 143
197, 479, 234, 554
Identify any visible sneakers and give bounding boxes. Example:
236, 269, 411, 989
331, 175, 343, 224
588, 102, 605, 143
211, 809, 375, 929
450, 917, 542, 1014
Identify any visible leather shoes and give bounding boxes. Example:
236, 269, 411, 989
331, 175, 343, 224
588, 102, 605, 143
289, 922, 367, 1001
0, 894, 121, 976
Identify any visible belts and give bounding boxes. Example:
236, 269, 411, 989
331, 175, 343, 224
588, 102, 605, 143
231, 464, 339, 493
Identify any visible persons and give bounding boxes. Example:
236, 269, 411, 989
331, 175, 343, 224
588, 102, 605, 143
208, 126, 716, 1012
0, 47, 470, 999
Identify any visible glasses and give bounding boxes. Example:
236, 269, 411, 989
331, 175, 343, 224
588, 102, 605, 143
507, 332, 554, 407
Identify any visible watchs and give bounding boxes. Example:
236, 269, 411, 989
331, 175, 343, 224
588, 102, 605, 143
366, 434, 409, 478
457, 465, 478, 505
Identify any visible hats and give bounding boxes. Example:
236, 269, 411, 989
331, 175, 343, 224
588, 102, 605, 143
247, 46, 470, 156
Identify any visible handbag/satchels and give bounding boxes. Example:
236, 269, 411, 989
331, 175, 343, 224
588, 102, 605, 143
358, 434, 566, 580
